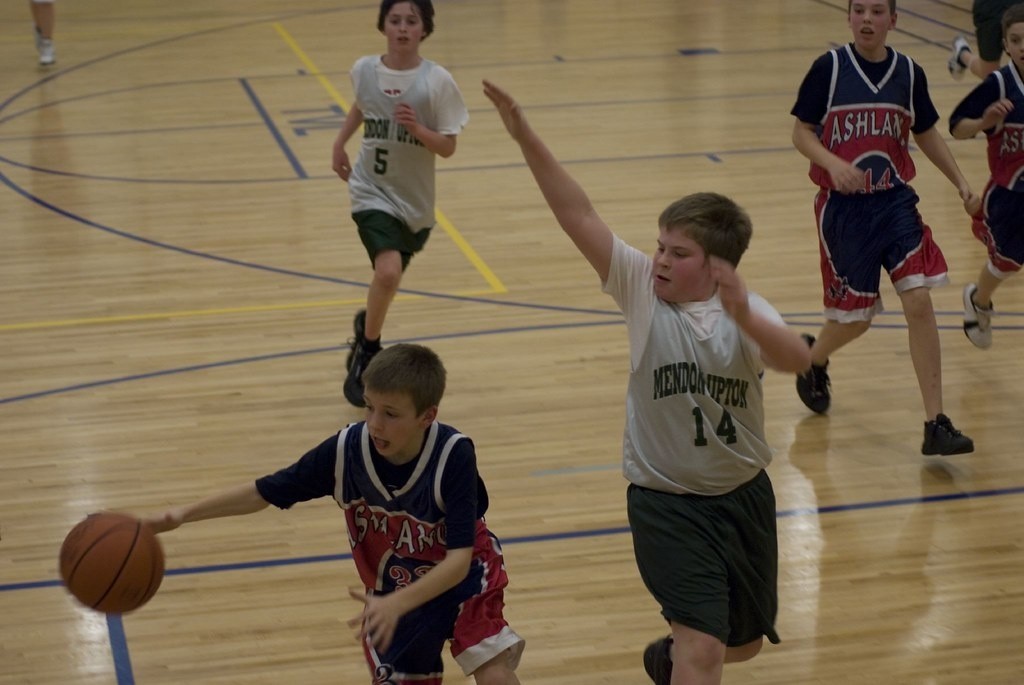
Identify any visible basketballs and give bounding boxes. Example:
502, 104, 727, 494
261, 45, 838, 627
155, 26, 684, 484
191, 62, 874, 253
59, 512, 164, 614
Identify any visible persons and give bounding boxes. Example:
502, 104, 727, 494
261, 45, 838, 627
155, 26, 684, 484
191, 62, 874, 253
947, 0, 1022, 82
477, 78, 811, 685
29, 0, 56, 65
144, 343, 526, 685
789, 1, 980, 457
947, 3, 1024, 351
333, 1, 469, 408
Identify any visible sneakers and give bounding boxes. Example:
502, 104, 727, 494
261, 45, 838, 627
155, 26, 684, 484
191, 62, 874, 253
948, 37, 972, 81
962, 284, 995, 346
345, 345, 381, 408
793, 334, 829, 413
347, 311, 366, 370
922, 413, 973, 455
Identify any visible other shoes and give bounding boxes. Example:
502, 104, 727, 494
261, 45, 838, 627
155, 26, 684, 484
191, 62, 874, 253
642, 638, 674, 685
31, 24, 41, 47
40, 38, 55, 63
